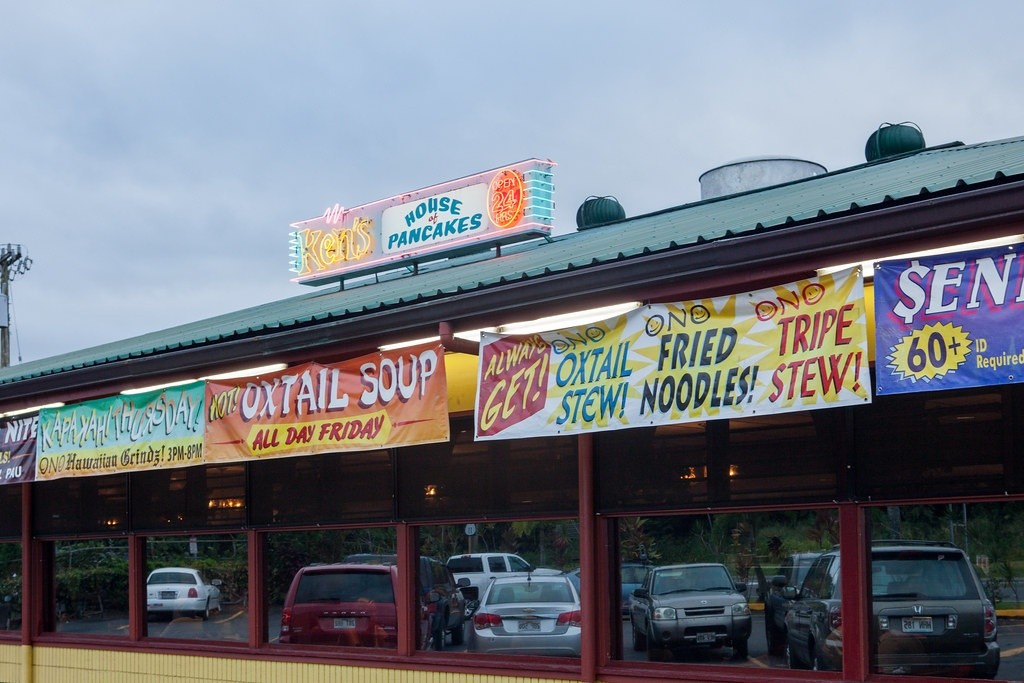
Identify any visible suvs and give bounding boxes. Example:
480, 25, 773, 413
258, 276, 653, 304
445, 552, 566, 622
336, 552, 471, 648
630, 564, 751, 664
778, 538, 1002, 680
278, 560, 448, 650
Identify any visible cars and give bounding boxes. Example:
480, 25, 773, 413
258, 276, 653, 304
562, 562, 659, 617
146, 567, 221, 621
470, 570, 582, 659
763, 554, 828, 662
